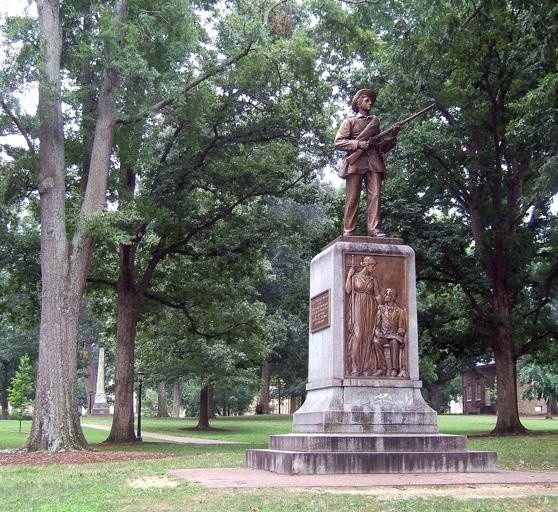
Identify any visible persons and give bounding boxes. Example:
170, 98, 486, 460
335, 87, 404, 240
369, 286, 407, 375
343, 256, 382, 378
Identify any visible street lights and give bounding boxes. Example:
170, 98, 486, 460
277, 381, 282, 413
136, 367, 144, 443
89, 390, 93, 415
